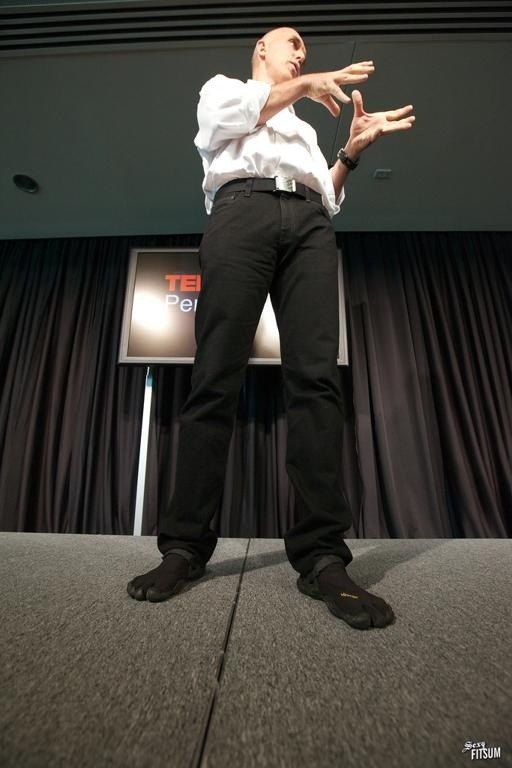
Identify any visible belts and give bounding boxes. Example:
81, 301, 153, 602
212, 175, 323, 204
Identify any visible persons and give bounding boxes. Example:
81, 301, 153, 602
126, 27, 419, 629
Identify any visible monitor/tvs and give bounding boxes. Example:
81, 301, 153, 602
114, 246, 352, 368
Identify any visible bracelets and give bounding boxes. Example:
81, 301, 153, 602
337, 148, 360, 172
